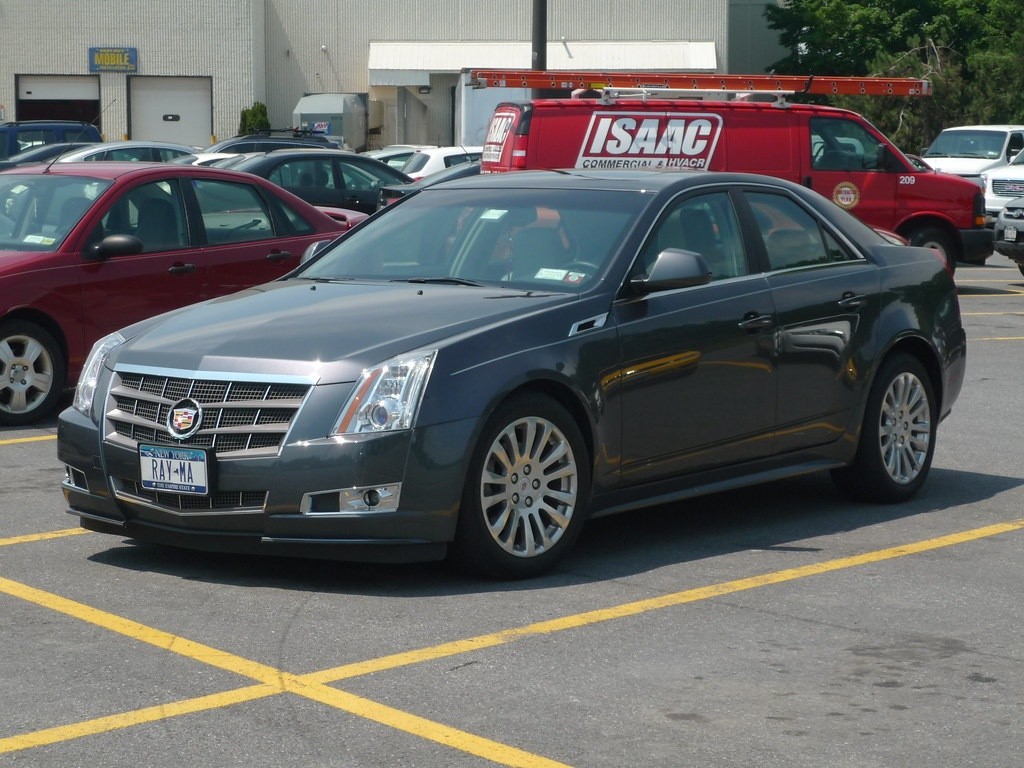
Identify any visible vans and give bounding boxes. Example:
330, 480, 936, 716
480, 99, 996, 278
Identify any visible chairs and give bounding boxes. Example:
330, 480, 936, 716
295, 173, 314, 187
765, 228, 818, 270
315, 172, 329, 188
647, 208, 728, 280
54, 196, 105, 244
511, 227, 566, 285
137, 199, 180, 245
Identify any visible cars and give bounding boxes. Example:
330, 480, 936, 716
0, 163, 370, 428
980, 148, 1024, 229
923, 125, 1024, 180
905, 154, 933, 171
811, 134, 866, 164
0, 120, 201, 178
224, 148, 416, 215
162, 153, 241, 174
994, 196, 1024, 276
55, 161, 969, 578
203, 129, 342, 155
209, 152, 268, 169
399, 145, 484, 181
359, 144, 443, 171
